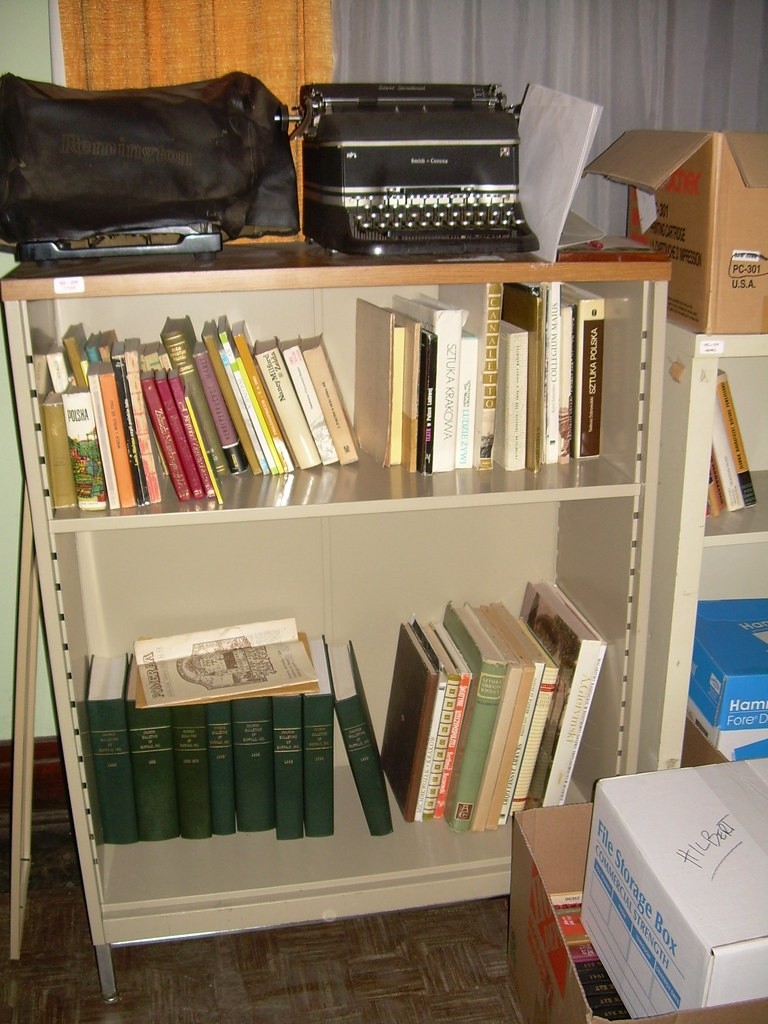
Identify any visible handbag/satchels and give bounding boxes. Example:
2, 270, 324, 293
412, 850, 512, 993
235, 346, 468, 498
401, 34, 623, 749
0, 71, 301, 244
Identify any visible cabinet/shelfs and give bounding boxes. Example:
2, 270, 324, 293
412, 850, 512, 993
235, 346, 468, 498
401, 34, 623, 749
1, 236, 676, 1007
639, 323, 767, 775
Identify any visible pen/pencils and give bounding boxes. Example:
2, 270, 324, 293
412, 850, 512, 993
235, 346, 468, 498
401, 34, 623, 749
586, 241, 604, 249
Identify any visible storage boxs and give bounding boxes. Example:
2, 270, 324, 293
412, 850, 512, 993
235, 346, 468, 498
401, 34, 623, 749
581, 757, 768, 1017
581, 128, 768, 333
685, 598, 767, 763
506, 803, 768, 1023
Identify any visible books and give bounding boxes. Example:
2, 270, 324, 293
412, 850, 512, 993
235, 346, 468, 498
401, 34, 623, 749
704, 370, 757, 517
45, 314, 358, 509
86, 619, 336, 845
325, 641, 392, 836
354, 282, 606, 476
381, 580, 609, 831
